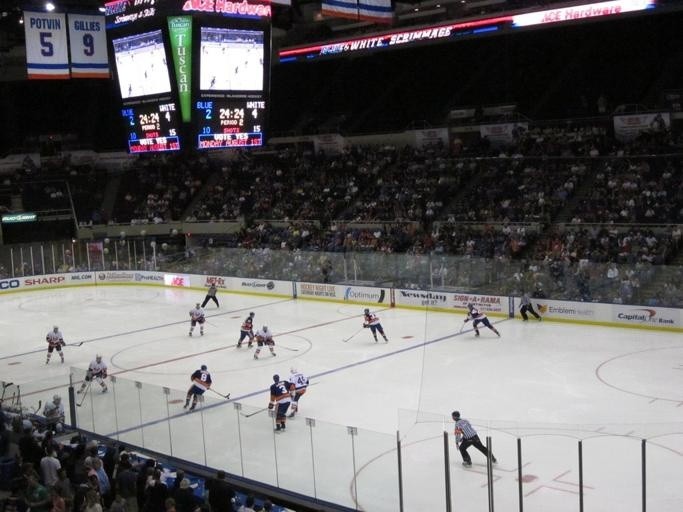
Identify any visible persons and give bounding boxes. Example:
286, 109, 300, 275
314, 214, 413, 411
268, 375, 296, 432
403, 124, 681, 309
518, 290, 542, 320
362, 308, 389, 343
1, 394, 279, 512
1, 123, 430, 289
450, 411, 499, 469
252, 324, 276, 360
463, 303, 501, 339
186, 302, 206, 337
77, 355, 108, 395
236, 311, 256, 349
288, 366, 310, 418
44, 324, 67, 364
182, 365, 212, 410
201, 283, 219, 308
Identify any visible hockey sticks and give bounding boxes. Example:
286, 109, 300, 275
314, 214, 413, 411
60, 342, 82, 346
207, 388, 230, 398
76, 379, 91, 407
239, 408, 268, 417
342, 327, 365, 342
